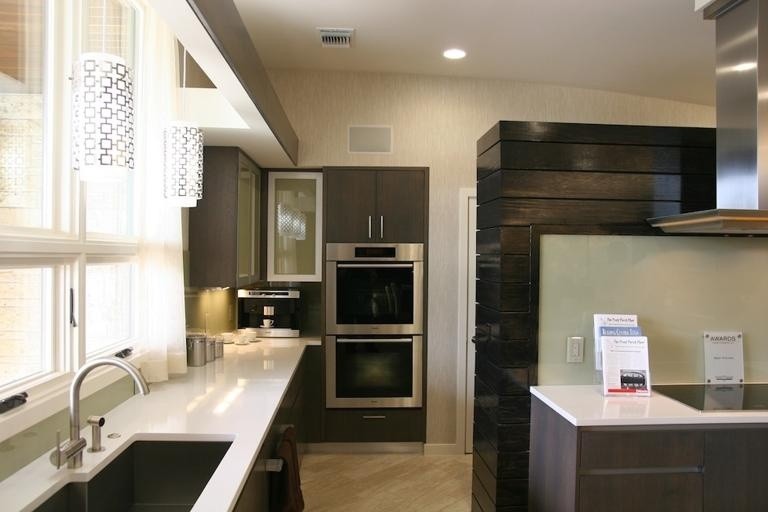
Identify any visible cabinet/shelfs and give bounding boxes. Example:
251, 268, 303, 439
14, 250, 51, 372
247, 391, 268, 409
190, 146, 262, 289
324, 165, 428, 245
321, 408, 426, 455
528, 396, 705, 512
281, 350, 306, 472
262, 167, 324, 283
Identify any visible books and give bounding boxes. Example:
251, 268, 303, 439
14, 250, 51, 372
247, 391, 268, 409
703, 330, 745, 383
703, 385, 744, 411
591, 311, 653, 397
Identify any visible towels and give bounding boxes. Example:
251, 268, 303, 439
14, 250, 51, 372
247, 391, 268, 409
269, 429, 305, 512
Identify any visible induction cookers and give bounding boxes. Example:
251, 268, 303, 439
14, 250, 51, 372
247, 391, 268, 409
650, 382, 767, 412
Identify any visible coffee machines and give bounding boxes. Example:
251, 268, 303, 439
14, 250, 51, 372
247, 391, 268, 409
235, 285, 302, 339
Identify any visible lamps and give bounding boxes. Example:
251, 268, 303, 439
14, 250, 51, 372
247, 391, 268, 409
276, 190, 306, 242
164, 48, 203, 204
68, 1, 135, 183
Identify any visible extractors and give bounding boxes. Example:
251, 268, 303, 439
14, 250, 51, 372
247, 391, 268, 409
648, 0, 768, 237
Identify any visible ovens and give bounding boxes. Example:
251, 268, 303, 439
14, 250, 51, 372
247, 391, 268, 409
326, 242, 424, 408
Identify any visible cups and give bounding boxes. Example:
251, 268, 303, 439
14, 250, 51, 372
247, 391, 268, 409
187, 334, 223, 368
223, 331, 256, 345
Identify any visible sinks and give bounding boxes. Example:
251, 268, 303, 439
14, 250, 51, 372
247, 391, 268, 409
19, 433, 236, 512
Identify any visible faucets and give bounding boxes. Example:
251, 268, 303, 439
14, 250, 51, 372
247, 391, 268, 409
49, 355, 150, 469
